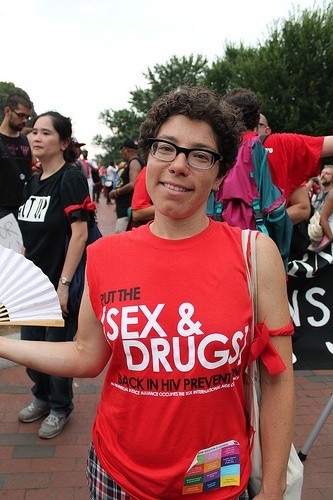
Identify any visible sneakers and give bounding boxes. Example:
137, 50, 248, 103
38, 414, 72, 438
17, 398, 51, 423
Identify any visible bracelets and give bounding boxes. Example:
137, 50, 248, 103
115, 188, 123, 197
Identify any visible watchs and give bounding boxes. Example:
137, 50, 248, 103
59, 276, 70, 287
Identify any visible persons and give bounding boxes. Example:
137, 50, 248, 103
0, 94, 35, 220
0, 88, 295, 500
304, 164, 333, 255
125, 163, 155, 232
253, 113, 312, 226
80, 148, 128, 206
15, 110, 96, 440
109, 139, 144, 234
205, 89, 333, 284
70, 136, 88, 180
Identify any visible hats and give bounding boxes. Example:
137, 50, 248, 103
120, 139, 138, 149
71, 136, 86, 147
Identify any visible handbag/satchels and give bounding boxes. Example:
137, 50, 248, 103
241, 229, 303, 500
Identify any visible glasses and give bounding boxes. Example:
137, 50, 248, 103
10, 107, 31, 120
146, 137, 223, 170
